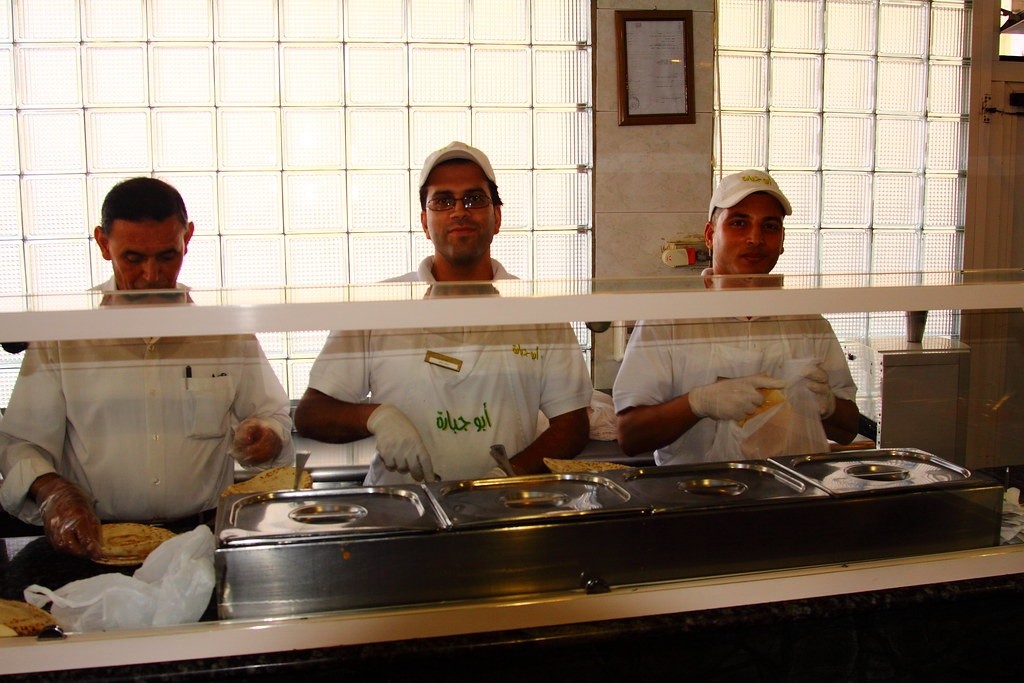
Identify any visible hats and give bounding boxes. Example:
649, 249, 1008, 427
708, 169, 792, 222
418, 142, 496, 195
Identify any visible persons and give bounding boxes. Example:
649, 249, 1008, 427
0, 176, 293, 559
293, 140, 594, 485
613, 168, 861, 467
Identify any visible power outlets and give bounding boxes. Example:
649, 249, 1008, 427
665, 240, 711, 269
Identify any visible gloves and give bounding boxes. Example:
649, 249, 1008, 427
791, 365, 836, 421
366, 403, 435, 485
687, 374, 786, 422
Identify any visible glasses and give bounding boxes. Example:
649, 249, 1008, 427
424, 193, 495, 211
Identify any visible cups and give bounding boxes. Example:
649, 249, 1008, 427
906, 310, 928, 343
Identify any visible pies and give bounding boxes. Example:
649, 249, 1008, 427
0, 598, 57, 635
221, 466, 312, 497
90, 523, 176, 565
543, 457, 633, 473
